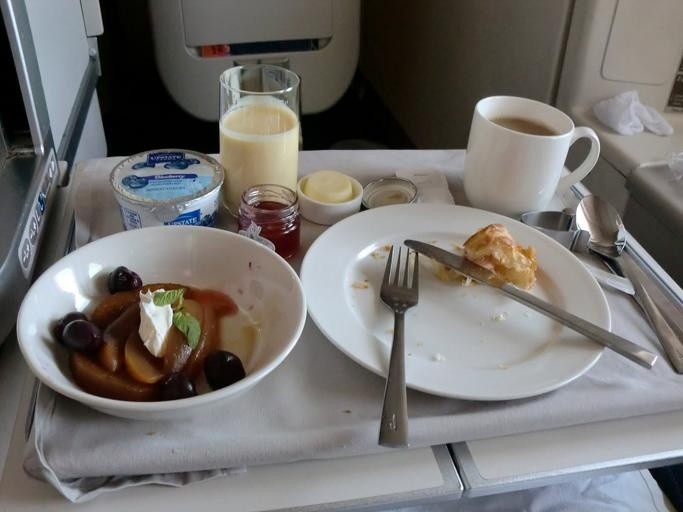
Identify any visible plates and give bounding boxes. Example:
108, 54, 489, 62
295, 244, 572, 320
300, 203, 613, 405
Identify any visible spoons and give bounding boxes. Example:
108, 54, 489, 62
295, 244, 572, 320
573, 197, 682, 373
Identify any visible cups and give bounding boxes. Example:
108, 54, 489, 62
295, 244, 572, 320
462, 96, 601, 218
218, 64, 301, 222
236, 182, 299, 259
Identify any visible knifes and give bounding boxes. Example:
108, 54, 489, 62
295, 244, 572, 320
404, 240, 656, 369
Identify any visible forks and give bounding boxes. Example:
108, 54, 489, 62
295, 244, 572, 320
375, 244, 420, 447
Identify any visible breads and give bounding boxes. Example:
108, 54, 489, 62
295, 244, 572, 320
439, 225, 536, 289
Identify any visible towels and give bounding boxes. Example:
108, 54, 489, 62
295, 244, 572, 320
591, 90, 674, 137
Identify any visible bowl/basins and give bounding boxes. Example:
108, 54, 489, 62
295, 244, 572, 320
14, 223, 306, 422
